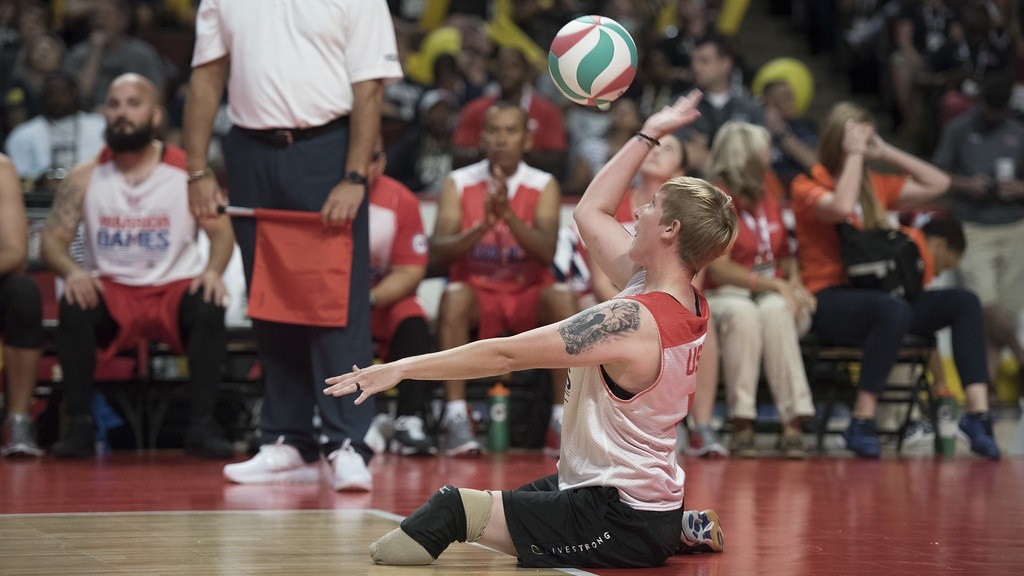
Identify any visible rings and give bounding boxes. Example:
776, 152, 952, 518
355, 383, 360, 392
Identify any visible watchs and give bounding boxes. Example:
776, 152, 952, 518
342, 171, 368, 186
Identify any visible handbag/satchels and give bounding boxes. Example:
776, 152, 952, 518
840, 231, 925, 297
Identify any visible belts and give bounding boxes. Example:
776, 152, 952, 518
237, 116, 349, 147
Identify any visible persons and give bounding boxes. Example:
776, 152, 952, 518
0, 0, 1024, 460
322, 90, 740, 568
183, 0, 403, 490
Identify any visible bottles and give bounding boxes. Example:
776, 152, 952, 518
485, 382, 510, 452
934, 386, 960, 458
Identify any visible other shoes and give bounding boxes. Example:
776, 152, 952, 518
727, 429, 760, 458
184, 416, 231, 456
846, 415, 882, 458
52, 415, 95, 456
960, 411, 997, 456
777, 436, 808, 459
542, 418, 562, 456
681, 420, 731, 457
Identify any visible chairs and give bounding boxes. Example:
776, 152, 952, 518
35, 330, 947, 457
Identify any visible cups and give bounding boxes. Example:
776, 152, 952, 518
995, 157, 1016, 189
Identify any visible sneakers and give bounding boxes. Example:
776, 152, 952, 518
389, 415, 437, 454
682, 509, 725, 551
1, 412, 41, 457
441, 410, 484, 458
223, 435, 320, 483
328, 438, 372, 492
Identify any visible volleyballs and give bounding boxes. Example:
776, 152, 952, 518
550, 15, 640, 106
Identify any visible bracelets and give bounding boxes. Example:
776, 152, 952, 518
187, 167, 212, 183
637, 131, 660, 146
